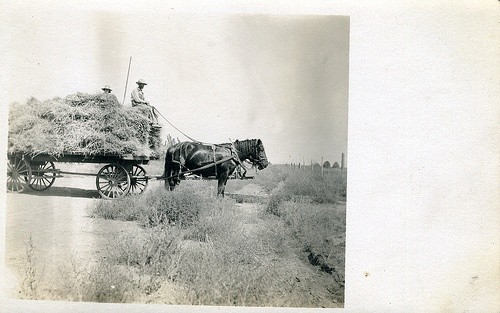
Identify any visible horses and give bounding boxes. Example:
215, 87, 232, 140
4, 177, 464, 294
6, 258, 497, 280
157, 138, 268, 199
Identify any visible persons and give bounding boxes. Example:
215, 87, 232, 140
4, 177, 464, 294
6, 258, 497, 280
130, 79, 162, 131
102, 85, 112, 95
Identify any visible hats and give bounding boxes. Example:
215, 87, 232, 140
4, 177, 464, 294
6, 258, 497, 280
136, 79, 147, 85
101, 85, 112, 91
